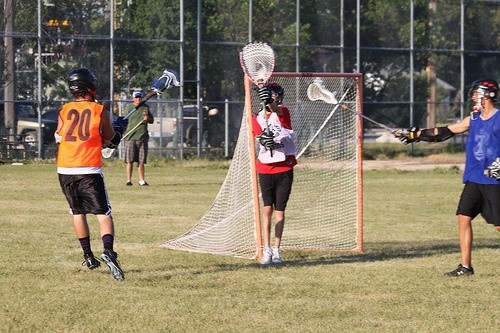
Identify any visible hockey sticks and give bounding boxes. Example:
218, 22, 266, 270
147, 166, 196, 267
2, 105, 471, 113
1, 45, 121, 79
121, 67, 180, 120
239, 42, 276, 157
100, 119, 143, 158
307, 83, 397, 133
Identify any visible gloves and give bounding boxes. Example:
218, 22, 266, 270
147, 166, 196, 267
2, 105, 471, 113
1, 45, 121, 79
391, 125, 420, 145
258, 87, 274, 105
259, 132, 284, 150
483, 161, 500, 181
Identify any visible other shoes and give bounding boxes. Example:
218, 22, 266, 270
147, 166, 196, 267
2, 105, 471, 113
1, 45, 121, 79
138, 181, 149, 186
126, 181, 133, 187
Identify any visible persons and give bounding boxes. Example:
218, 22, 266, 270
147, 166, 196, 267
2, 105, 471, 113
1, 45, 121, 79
55, 68, 129, 282
392, 78, 500, 277
123, 89, 154, 186
252, 82, 297, 265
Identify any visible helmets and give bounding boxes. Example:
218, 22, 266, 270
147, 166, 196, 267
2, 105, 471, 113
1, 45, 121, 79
68, 67, 97, 90
268, 83, 284, 103
471, 77, 499, 100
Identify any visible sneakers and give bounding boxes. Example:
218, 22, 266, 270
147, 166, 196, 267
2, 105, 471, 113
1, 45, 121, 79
271, 248, 282, 263
261, 248, 272, 265
100, 251, 124, 280
81, 252, 101, 269
444, 264, 474, 278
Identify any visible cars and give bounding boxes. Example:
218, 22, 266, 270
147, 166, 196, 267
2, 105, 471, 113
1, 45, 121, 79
42, 106, 128, 146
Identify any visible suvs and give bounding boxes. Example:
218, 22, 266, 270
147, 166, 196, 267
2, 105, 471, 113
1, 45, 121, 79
0, 99, 58, 151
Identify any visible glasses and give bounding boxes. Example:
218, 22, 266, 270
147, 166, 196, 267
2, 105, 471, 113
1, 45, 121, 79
134, 96, 142, 98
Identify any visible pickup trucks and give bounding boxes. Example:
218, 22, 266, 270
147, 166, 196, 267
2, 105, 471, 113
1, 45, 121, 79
147, 105, 225, 148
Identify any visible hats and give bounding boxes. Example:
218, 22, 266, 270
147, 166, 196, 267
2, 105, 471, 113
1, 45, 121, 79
133, 90, 143, 98
